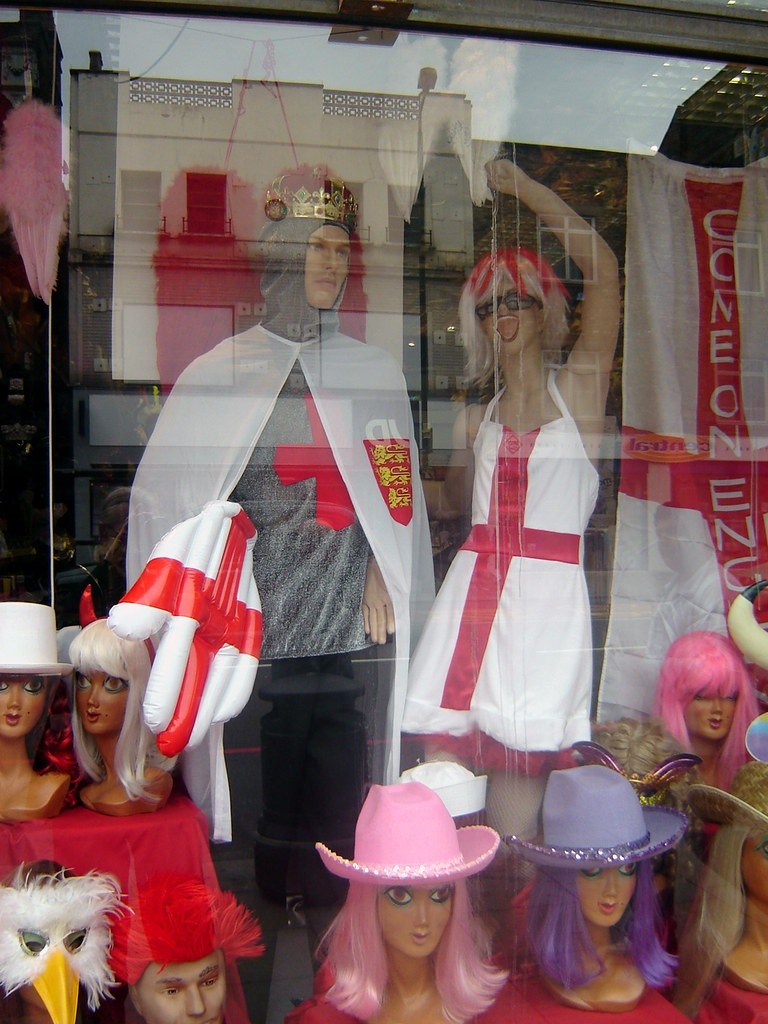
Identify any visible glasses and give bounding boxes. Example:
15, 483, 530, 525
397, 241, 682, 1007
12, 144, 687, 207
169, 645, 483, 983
475, 293, 542, 321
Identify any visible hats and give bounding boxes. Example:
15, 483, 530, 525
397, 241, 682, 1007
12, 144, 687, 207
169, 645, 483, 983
316, 782, 501, 886
503, 765, 688, 869
688, 762, 768, 836
0, 601, 75, 676
393, 761, 488, 816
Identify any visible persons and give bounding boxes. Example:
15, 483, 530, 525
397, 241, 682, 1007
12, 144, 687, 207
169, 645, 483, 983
0, 601, 74, 825
650, 631, 760, 845
672, 761, 768, 1024
68, 616, 173, 816
502, 765, 688, 1012
136, 211, 422, 1024
313, 780, 511, 1023
97, 486, 174, 566
400, 157, 622, 879
110, 873, 265, 1024
650, 499, 751, 669
0, 860, 129, 1024
